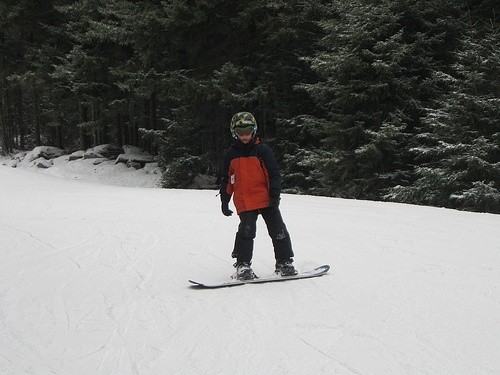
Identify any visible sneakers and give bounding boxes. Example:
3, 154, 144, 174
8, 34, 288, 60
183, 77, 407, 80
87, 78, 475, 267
275, 258, 298, 276
233, 261, 254, 279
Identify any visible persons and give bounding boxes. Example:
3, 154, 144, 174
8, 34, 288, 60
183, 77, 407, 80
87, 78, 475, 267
219, 111, 298, 280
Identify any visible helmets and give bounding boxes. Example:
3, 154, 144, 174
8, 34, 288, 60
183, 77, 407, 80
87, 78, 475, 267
229, 112, 257, 144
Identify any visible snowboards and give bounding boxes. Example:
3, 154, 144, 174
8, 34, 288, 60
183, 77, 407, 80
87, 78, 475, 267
188, 263, 331, 288
